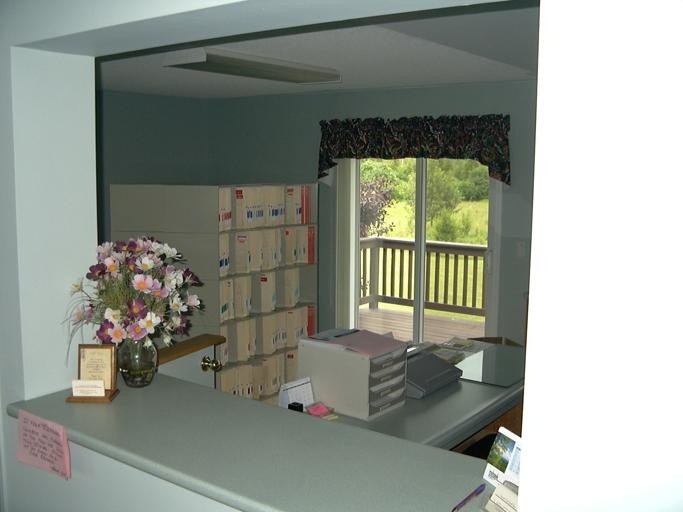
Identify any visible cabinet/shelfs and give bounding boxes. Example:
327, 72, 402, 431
299, 326, 409, 422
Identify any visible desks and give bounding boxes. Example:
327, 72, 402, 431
303, 337, 522, 459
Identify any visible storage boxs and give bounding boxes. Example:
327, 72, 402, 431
101, 173, 318, 407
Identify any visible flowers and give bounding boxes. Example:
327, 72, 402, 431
54, 227, 208, 375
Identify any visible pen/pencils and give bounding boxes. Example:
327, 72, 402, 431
451, 483, 486, 512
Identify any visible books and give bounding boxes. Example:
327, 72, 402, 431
405, 353, 464, 398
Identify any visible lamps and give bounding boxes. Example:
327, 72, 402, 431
158, 45, 342, 88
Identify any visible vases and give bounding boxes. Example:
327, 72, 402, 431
114, 336, 161, 388
